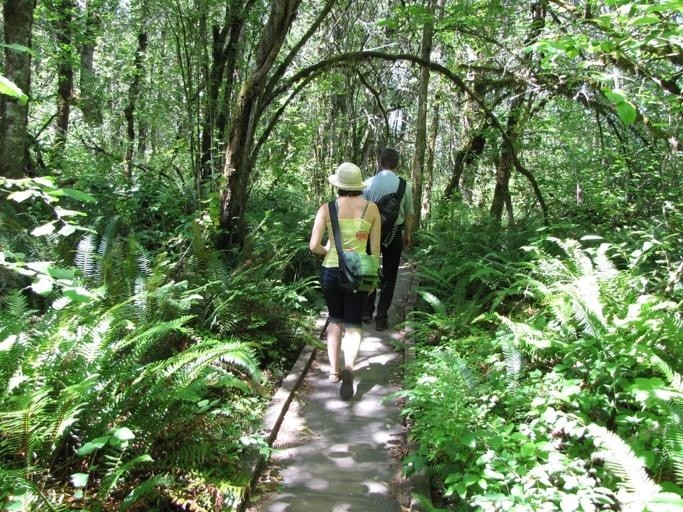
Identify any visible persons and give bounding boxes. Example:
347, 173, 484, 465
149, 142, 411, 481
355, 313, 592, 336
362, 148, 409, 331
309, 162, 382, 399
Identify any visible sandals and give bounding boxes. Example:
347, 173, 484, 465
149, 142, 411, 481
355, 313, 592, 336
329, 366, 354, 400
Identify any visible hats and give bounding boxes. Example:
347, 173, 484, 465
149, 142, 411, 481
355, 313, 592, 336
328, 162, 367, 192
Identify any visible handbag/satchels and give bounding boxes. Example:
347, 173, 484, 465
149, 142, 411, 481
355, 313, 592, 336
339, 253, 381, 293
378, 193, 400, 240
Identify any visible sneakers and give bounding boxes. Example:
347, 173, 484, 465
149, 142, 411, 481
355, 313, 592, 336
362, 312, 385, 331
